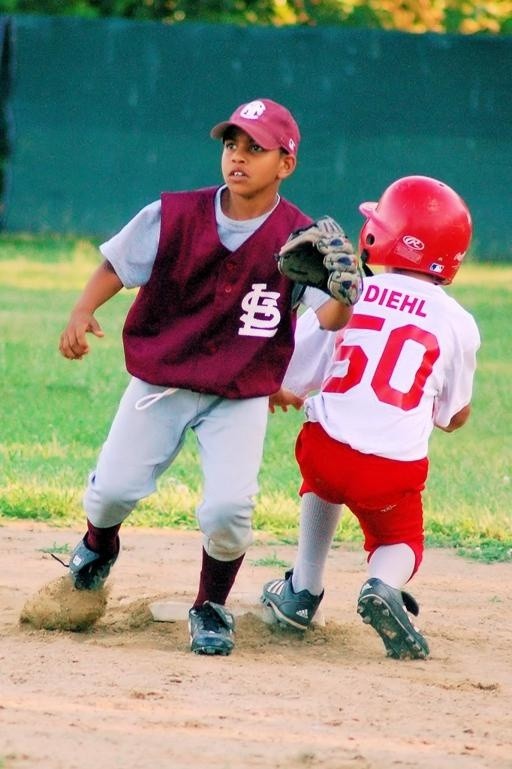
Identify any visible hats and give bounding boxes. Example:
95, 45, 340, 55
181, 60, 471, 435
210, 98, 301, 155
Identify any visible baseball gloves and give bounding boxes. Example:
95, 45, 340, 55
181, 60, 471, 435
271, 213, 365, 308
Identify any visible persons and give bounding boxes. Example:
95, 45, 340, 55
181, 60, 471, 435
59, 97, 364, 656
259, 175, 480, 664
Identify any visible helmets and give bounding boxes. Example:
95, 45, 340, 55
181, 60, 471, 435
358, 175, 473, 285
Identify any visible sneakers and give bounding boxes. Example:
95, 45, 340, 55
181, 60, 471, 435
260, 568, 324, 631
356, 578, 430, 661
69, 531, 121, 592
188, 600, 235, 656
